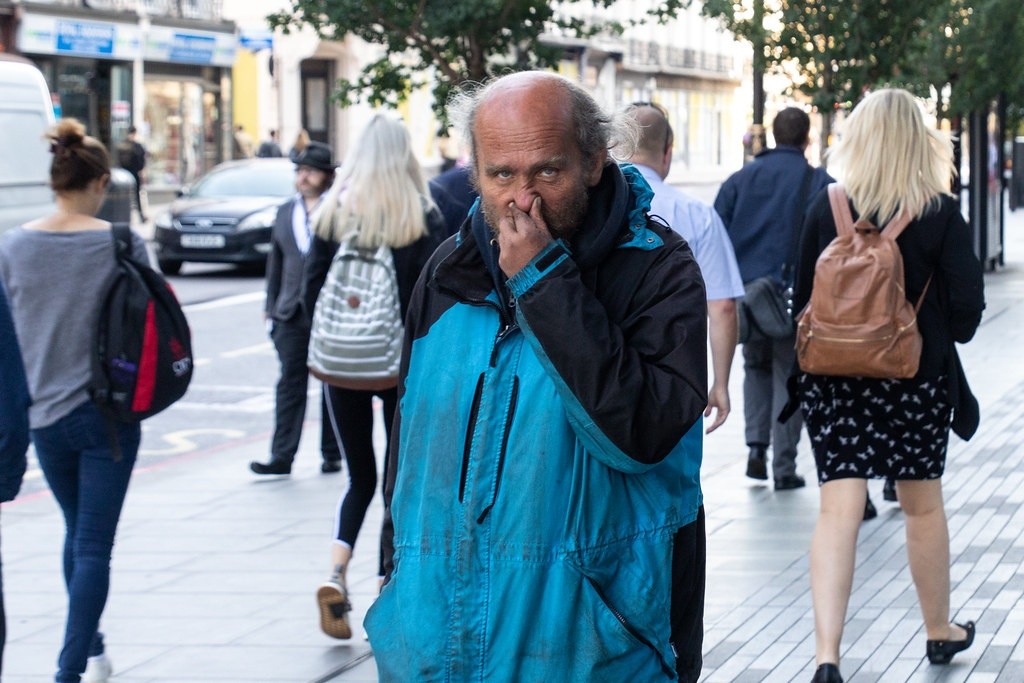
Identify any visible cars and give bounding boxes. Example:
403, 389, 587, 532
152, 156, 299, 277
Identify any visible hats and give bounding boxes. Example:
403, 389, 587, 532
290, 142, 342, 171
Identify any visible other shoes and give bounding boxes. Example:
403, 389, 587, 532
775, 474, 807, 488
322, 456, 341, 473
82, 653, 111, 683
810, 663, 843, 683
927, 620, 975, 665
249, 460, 290, 475
862, 493, 878, 520
317, 570, 351, 638
884, 476, 897, 502
747, 441, 769, 478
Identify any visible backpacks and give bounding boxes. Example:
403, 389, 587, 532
86, 222, 193, 424
796, 183, 933, 378
306, 232, 406, 391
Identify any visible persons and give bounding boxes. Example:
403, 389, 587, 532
234, 124, 283, 160
118, 126, 148, 224
288, 129, 311, 163
0, 117, 151, 683
778, 88, 986, 683
612, 102, 746, 683
712, 108, 838, 491
0, 279, 33, 683
863, 478, 897, 520
297, 110, 477, 639
363, 70, 707, 683
250, 141, 342, 475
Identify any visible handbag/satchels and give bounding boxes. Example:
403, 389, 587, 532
738, 276, 793, 341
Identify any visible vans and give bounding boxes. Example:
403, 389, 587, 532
0, 59, 61, 240
